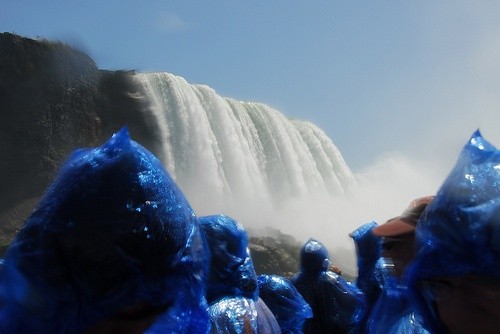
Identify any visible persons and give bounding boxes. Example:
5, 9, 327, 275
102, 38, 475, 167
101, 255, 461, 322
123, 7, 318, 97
0, 123, 500, 334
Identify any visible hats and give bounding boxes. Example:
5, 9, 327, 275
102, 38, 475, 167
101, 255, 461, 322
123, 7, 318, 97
372, 196, 435, 236
